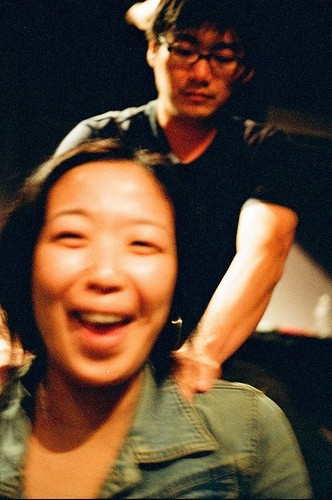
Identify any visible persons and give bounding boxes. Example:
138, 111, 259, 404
0, 139, 311, 500
50, 0, 305, 403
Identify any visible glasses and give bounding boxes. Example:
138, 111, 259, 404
158, 35, 246, 78
